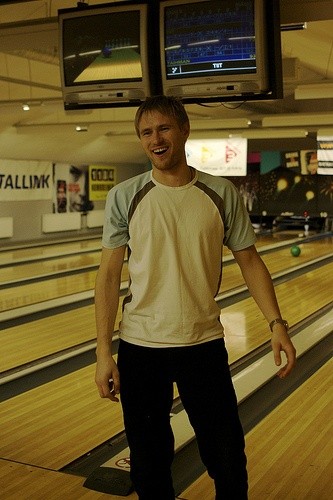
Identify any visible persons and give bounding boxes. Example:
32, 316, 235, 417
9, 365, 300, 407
94, 93, 298, 500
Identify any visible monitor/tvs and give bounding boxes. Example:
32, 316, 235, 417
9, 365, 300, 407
58, 4, 151, 105
159, 0, 269, 99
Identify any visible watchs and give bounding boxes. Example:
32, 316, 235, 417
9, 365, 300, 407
268, 318, 288, 330
